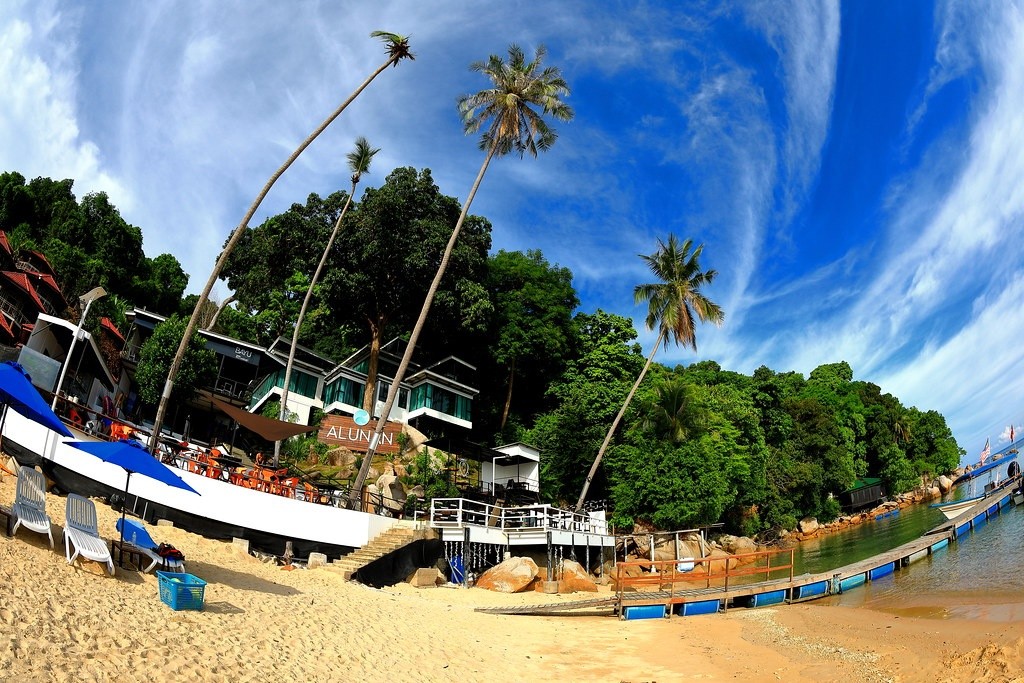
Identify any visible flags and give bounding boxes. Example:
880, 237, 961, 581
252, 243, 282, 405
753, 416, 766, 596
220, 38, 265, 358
1010, 426, 1014, 443
980, 438, 990, 463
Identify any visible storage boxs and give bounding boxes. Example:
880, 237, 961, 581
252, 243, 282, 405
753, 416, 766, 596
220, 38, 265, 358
156, 570, 206, 610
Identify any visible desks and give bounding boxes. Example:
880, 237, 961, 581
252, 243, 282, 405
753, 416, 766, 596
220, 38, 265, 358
312, 484, 341, 507
210, 458, 244, 484
159, 440, 190, 468
112, 539, 143, 571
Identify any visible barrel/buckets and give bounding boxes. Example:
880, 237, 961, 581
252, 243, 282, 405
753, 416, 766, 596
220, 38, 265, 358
748, 590, 786, 606
624, 605, 666, 620
876, 509, 898, 520
840, 573, 865, 591
905, 548, 928, 565
956, 522, 970, 537
1001, 495, 1010, 506
871, 561, 895, 581
973, 512, 986, 525
987, 504, 998, 517
680, 600, 720, 616
931, 539, 948, 552
794, 581, 828, 599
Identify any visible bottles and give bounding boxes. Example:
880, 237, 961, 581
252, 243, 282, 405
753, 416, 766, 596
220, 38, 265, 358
132, 532, 136, 546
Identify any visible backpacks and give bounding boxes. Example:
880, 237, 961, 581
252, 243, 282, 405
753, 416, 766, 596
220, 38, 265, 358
152, 542, 183, 560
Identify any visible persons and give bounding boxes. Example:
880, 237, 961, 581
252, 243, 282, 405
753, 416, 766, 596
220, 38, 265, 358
255, 450, 265, 465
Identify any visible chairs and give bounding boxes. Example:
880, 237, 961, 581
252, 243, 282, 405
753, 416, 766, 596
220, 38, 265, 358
185, 447, 326, 504
117, 517, 185, 575
7, 466, 55, 548
506, 479, 530, 490
69, 408, 138, 445
62, 494, 116, 576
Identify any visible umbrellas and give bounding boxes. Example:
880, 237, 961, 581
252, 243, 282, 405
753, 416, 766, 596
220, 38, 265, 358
61, 436, 201, 547
0, 360, 75, 439
